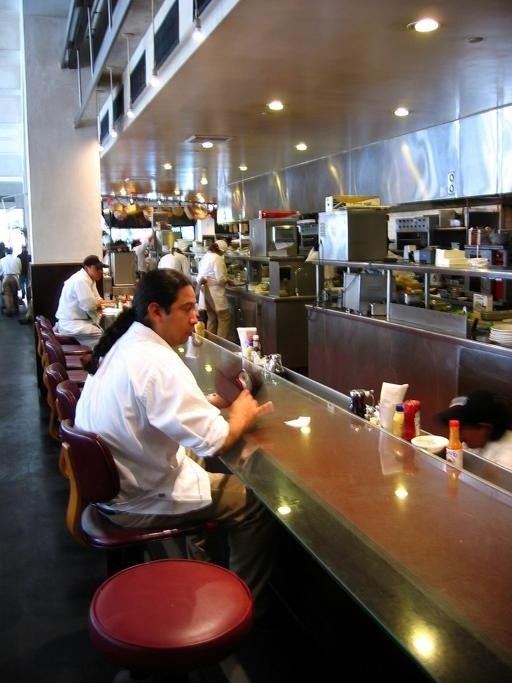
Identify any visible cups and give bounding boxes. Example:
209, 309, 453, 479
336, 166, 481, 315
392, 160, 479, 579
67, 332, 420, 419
348, 389, 375, 418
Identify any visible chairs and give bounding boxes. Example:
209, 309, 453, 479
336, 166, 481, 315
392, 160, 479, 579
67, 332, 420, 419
42, 340, 88, 383
39, 327, 85, 368
54, 379, 81, 479
58, 417, 231, 567
34, 319, 89, 355
34, 314, 78, 344
43, 362, 69, 439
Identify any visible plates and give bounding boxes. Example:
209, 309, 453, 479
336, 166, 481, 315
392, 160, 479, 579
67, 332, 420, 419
488, 323, 512, 347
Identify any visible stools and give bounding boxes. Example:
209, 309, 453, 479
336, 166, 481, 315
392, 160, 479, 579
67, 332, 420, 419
88, 557, 254, 683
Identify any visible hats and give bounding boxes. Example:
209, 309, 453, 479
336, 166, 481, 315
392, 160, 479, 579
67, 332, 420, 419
174, 239, 190, 253
434, 391, 504, 427
215, 240, 228, 253
83, 256, 110, 268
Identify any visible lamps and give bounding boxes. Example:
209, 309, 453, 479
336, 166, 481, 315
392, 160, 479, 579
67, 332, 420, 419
265, 100, 284, 111
392, 106, 411, 118
407, 18, 442, 34
295, 143, 307, 152
201, 141, 214, 149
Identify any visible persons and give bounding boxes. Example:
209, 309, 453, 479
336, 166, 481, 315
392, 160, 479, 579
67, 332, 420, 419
157, 239, 191, 282
130, 232, 153, 280
196, 240, 233, 340
432, 390, 512, 474
55, 255, 110, 351
74, 269, 277, 610
0, 242, 31, 318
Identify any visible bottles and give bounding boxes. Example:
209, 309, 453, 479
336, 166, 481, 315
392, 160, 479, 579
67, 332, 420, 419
447, 420, 464, 469
393, 404, 404, 435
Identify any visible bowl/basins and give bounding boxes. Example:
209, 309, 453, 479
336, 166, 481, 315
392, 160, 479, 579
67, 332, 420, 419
410, 434, 449, 454
